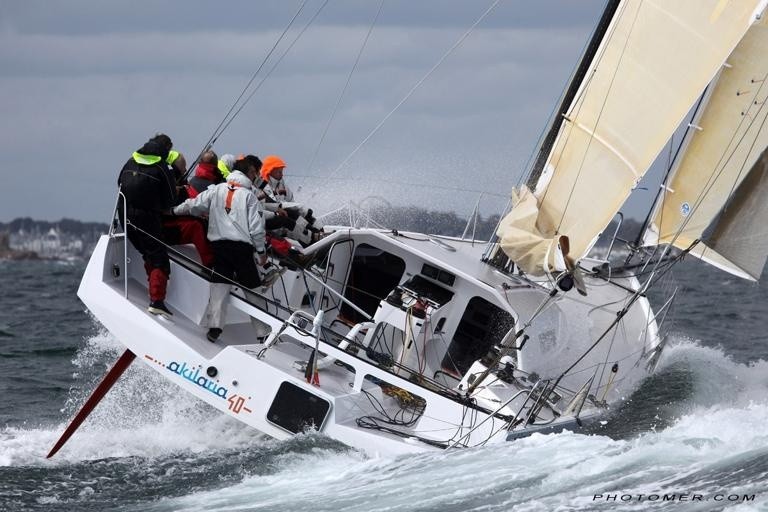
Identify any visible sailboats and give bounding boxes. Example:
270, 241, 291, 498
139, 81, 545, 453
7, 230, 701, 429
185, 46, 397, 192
46, 2, 768, 463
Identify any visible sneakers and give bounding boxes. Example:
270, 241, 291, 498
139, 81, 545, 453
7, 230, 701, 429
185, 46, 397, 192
207, 329, 220, 343
148, 300, 173, 318
